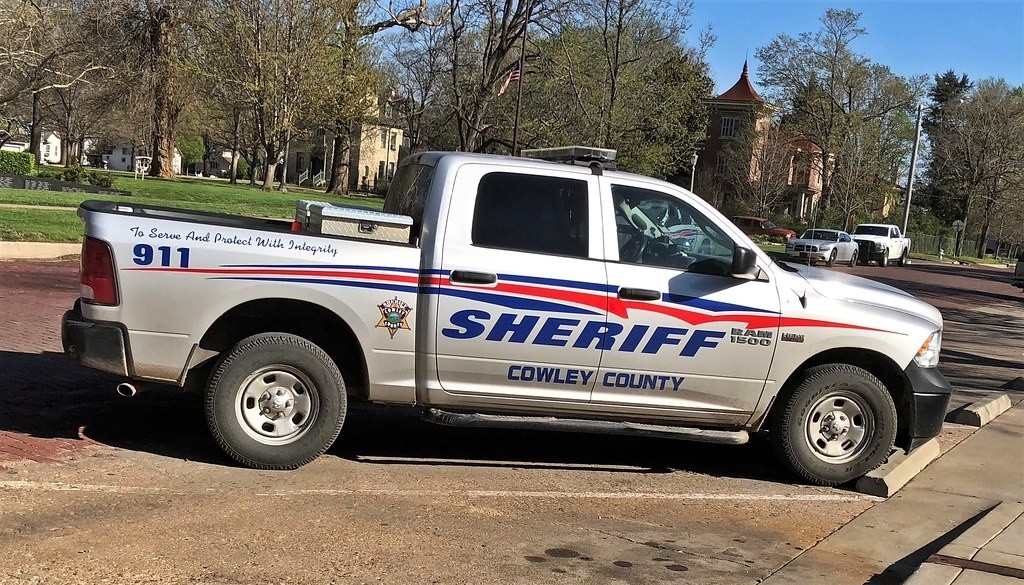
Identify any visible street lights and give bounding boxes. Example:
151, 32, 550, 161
903, 98, 964, 238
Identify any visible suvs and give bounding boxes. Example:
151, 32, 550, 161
732, 216, 796, 243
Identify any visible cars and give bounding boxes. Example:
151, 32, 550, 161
785, 229, 859, 267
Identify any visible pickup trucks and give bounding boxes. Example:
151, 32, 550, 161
62, 145, 945, 487
849, 224, 911, 267
